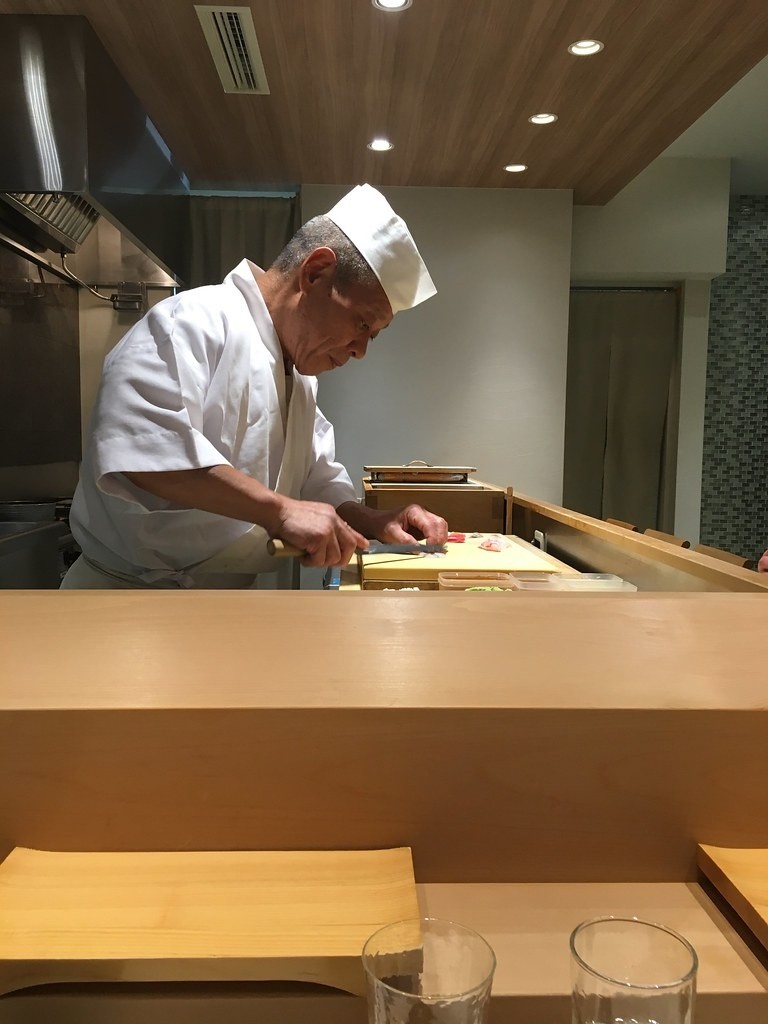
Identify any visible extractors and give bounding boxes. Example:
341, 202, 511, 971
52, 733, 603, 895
0, 190, 100, 254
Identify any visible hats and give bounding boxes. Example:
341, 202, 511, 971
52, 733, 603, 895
326, 182, 438, 316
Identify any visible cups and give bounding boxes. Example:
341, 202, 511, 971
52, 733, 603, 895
362, 917, 498, 1024
570, 915, 698, 1024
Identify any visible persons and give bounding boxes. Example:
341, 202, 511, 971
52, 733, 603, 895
56, 179, 447, 591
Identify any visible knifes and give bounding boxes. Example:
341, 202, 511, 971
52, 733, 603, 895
267, 539, 447, 558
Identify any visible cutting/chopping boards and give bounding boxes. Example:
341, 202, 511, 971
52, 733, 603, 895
358, 532, 562, 580
